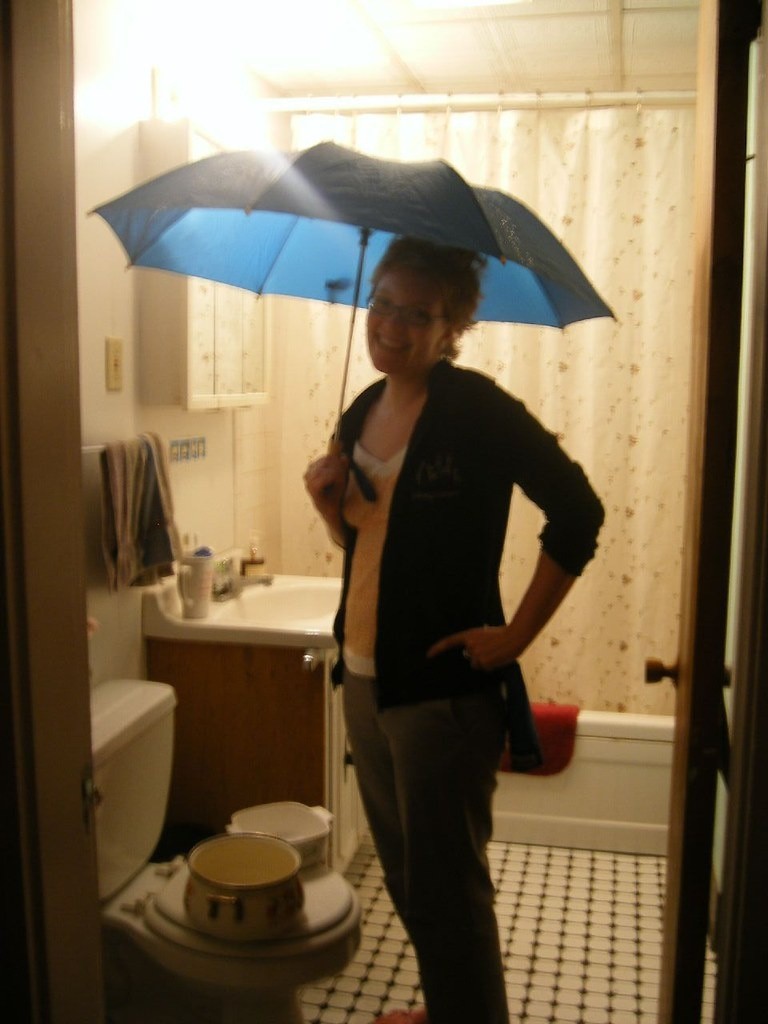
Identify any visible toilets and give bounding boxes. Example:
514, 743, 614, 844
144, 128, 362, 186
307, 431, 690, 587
88, 676, 361, 1023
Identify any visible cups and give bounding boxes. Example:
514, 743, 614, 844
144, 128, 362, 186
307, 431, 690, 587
178, 547, 213, 618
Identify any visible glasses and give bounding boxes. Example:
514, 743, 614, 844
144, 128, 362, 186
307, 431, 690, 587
366, 294, 450, 327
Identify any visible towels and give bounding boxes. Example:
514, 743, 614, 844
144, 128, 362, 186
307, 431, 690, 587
100, 431, 185, 594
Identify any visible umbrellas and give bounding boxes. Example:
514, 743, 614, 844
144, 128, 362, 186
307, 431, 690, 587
85, 141, 619, 499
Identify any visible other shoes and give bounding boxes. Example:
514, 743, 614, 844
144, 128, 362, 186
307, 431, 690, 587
373, 1011, 429, 1024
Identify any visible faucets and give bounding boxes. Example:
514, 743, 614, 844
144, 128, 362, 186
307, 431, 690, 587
210, 555, 271, 601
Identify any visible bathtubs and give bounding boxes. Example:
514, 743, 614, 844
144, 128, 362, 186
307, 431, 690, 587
490, 702, 673, 857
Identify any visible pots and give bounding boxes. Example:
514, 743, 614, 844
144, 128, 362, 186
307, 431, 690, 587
225, 801, 336, 870
184, 832, 305, 942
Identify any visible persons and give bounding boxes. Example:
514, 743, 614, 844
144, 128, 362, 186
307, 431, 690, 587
302, 230, 606, 1024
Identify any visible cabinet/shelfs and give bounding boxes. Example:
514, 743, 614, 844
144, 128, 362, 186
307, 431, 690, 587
145, 636, 372, 873
136, 118, 275, 412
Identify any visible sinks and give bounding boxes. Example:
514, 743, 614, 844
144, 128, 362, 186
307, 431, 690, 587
200, 571, 343, 650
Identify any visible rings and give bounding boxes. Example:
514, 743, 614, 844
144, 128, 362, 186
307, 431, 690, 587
462, 649, 470, 659
